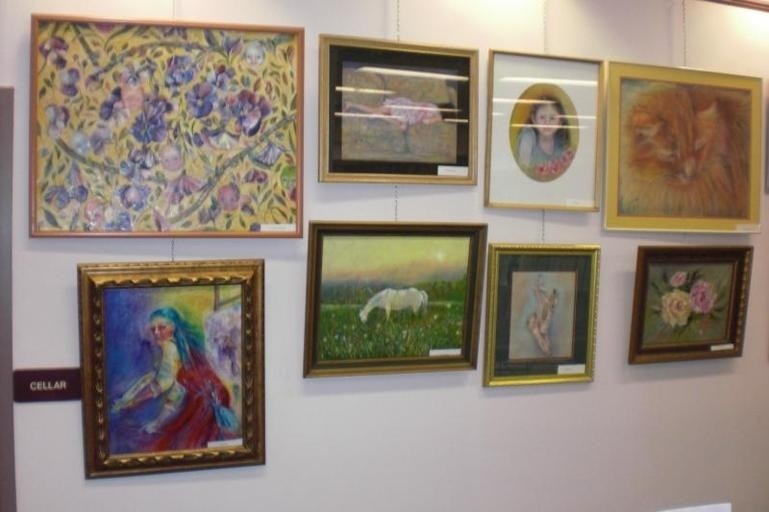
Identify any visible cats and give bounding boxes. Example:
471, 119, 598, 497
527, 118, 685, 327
619, 81, 748, 219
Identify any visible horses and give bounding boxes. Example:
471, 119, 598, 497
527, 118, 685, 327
357, 286, 429, 324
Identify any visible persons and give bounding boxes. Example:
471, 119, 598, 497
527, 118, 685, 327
114, 306, 230, 453
516, 95, 571, 172
342, 97, 457, 132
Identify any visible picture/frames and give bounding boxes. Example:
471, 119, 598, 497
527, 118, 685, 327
603, 61, 762, 233
484, 48, 605, 212
629, 244, 754, 365
77, 259, 265, 478
29, 14, 304, 238
317, 34, 479, 185
485, 242, 601, 386
302, 220, 487, 379
